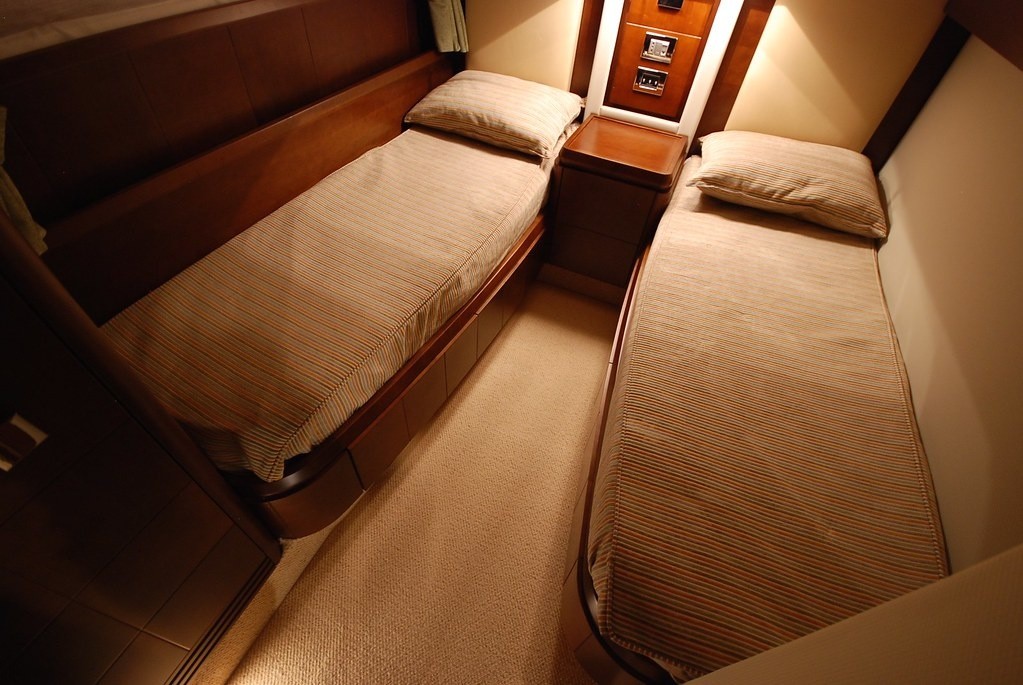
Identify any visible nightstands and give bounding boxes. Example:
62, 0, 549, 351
534, 111, 687, 307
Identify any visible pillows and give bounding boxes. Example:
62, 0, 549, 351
402, 68, 584, 157
698, 131, 890, 243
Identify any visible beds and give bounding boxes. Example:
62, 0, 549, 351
569, 155, 951, 675
96, 121, 589, 538
